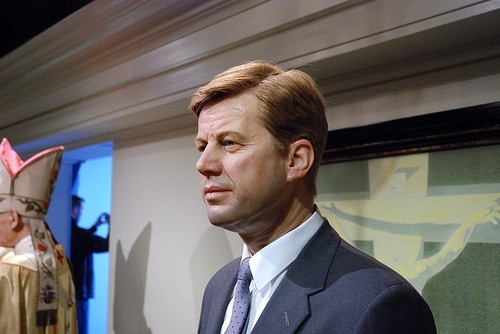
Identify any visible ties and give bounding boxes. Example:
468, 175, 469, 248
224, 257, 254, 334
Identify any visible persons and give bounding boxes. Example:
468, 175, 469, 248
0, 137, 79, 334
189, 61, 436, 334
70, 194, 109, 334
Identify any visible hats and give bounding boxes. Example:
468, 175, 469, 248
0, 138, 65, 219
